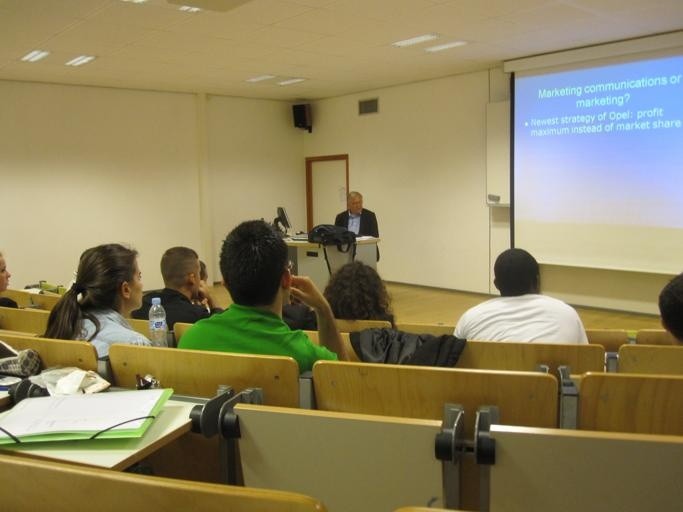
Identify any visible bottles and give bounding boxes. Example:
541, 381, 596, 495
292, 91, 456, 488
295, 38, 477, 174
147, 297, 167, 346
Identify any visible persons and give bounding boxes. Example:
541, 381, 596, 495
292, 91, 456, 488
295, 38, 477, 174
324, 261, 396, 332
659, 273, 682, 346
178, 220, 352, 378
282, 266, 319, 330
454, 249, 592, 343
132, 246, 225, 332
335, 191, 380, 261
193, 260, 209, 313
1, 252, 18, 308
41, 243, 152, 379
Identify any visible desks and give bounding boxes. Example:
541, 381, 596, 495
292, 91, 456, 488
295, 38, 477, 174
2, 386, 210, 471
280, 234, 382, 316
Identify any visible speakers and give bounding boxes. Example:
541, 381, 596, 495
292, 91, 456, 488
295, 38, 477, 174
292, 104, 312, 130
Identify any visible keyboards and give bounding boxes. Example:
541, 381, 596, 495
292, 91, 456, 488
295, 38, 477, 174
296, 230, 304, 235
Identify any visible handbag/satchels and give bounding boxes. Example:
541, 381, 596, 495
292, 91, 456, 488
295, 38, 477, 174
308, 224, 357, 275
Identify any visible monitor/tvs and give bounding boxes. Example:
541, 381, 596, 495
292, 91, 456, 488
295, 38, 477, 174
272, 206, 291, 238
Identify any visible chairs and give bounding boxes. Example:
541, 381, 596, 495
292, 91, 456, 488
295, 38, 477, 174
395, 323, 457, 339
576, 371, 681, 436
453, 341, 606, 379
634, 329, 681, 345
615, 344, 681, 376
2, 452, 327, 508
109, 343, 302, 410
2, 335, 98, 376
310, 360, 561, 431
2, 285, 392, 362
585, 328, 628, 353
